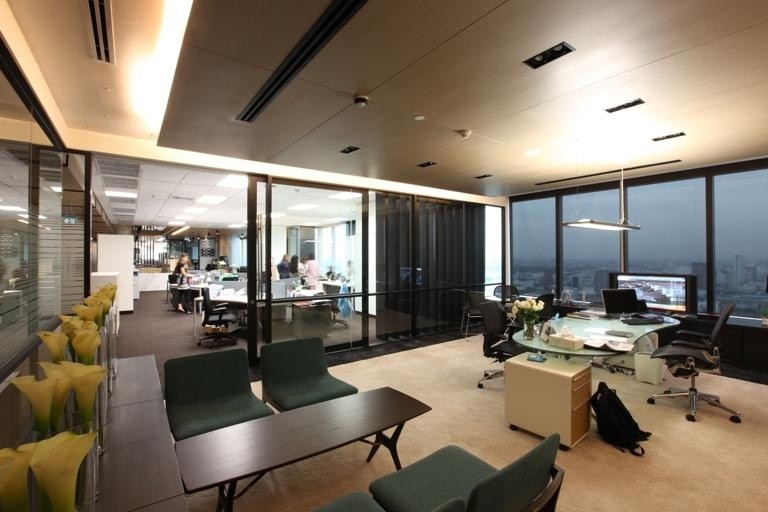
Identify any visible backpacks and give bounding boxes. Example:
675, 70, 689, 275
588, 381, 641, 449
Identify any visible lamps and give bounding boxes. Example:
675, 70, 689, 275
563, 170, 640, 231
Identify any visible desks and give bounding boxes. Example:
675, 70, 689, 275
513, 315, 680, 356
174, 386, 432, 512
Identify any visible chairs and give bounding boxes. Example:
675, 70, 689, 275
318, 433, 564, 512
647, 303, 741, 424
260, 336, 358, 412
163, 348, 274, 443
460, 286, 554, 389
167, 265, 349, 351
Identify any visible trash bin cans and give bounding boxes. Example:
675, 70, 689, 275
633, 351, 665, 385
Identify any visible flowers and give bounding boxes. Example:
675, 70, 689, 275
0, 284, 117, 512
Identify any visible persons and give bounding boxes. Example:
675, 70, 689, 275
277, 253, 337, 290
172, 254, 192, 313
223, 265, 233, 274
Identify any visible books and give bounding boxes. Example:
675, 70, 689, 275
568, 310, 601, 320
604, 329, 634, 339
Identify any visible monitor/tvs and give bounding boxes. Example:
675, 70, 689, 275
601, 288, 639, 315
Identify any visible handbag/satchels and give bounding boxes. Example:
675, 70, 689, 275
168, 275, 183, 284
